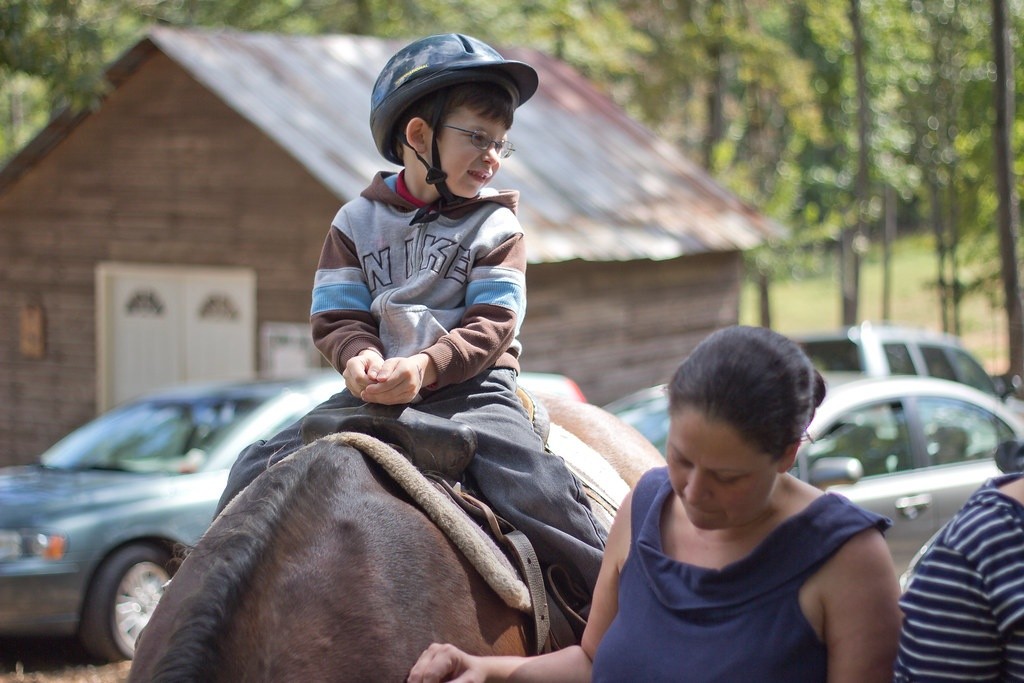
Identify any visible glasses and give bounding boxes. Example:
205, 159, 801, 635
429, 119, 516, 160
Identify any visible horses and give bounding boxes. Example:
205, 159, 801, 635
121, 389, 670, 683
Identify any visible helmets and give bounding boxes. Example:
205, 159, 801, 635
369, 33, 540, 168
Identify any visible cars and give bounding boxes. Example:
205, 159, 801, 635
602, 324, 996, 526
0, 367, 605, 668
782, 374, 1023, 595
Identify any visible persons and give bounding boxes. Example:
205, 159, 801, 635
895, 467, 1024, 683
403, 325, 902, 683
211, 34, 609, 642
